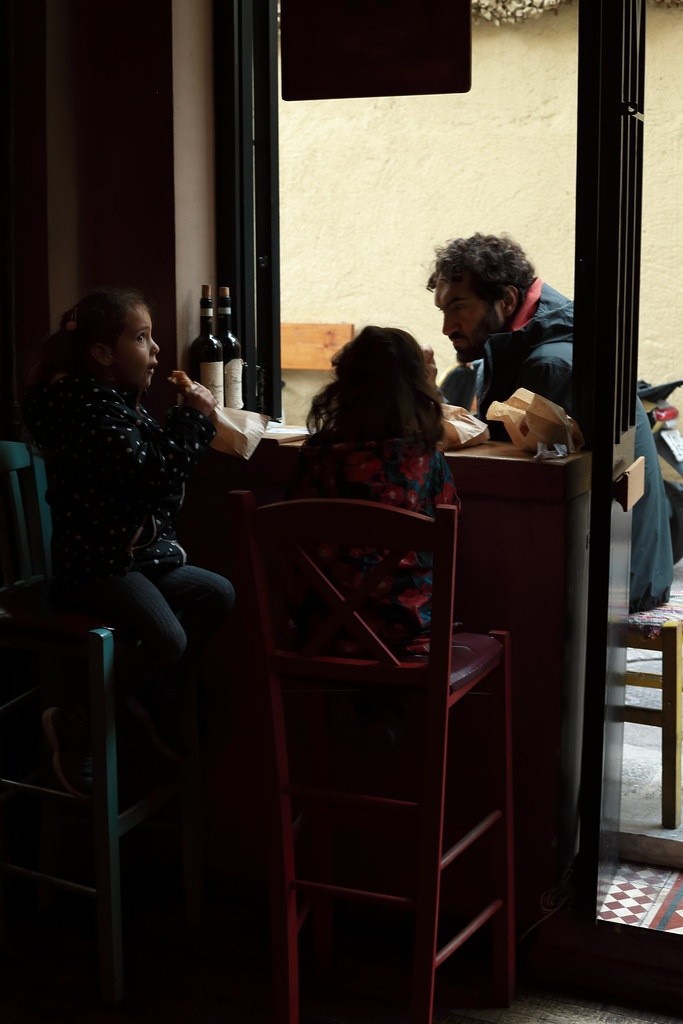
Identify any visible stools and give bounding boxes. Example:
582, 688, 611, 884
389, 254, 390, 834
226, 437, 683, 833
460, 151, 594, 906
1, 442, 162, 995
229, 488, 517, 1023
625, 599, 683, 830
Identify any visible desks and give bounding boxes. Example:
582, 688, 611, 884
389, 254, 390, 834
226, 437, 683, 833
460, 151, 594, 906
211, 413, 636, 926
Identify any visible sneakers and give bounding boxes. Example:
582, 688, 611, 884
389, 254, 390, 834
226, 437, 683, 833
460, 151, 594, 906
41, 706, 96, 800
125, 692, 194, 765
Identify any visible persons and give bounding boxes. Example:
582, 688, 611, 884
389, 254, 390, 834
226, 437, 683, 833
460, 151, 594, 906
19, 285, 237, 799
285, 326, 462, 757
428, 237, 672, 613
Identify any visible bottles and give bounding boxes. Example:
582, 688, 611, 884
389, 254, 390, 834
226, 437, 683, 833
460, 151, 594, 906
186, 284, 224, 408
216, 287, 244, 411
